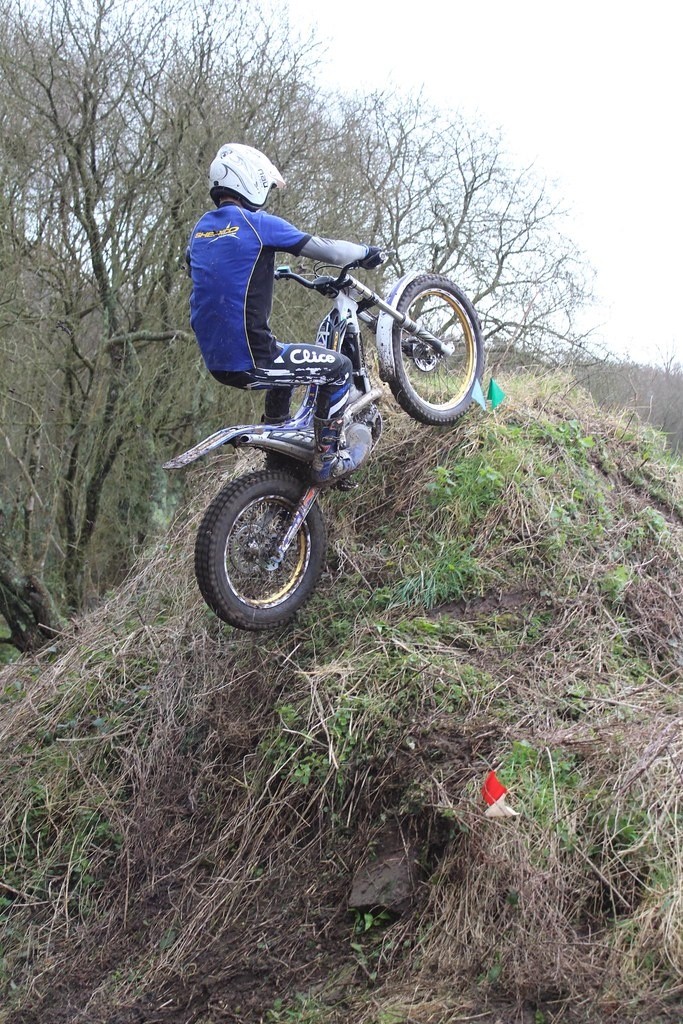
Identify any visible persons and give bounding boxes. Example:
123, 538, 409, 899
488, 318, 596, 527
183, 143, 388, 483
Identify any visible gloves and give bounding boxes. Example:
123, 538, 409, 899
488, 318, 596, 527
366, 246, 383, 270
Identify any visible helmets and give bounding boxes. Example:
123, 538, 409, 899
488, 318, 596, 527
210, 143, 286, 211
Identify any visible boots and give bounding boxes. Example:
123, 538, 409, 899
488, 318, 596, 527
260, 415, 291, 469
311, 415, 372, 484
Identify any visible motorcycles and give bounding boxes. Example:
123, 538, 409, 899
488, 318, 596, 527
162, 251, 486, 632
